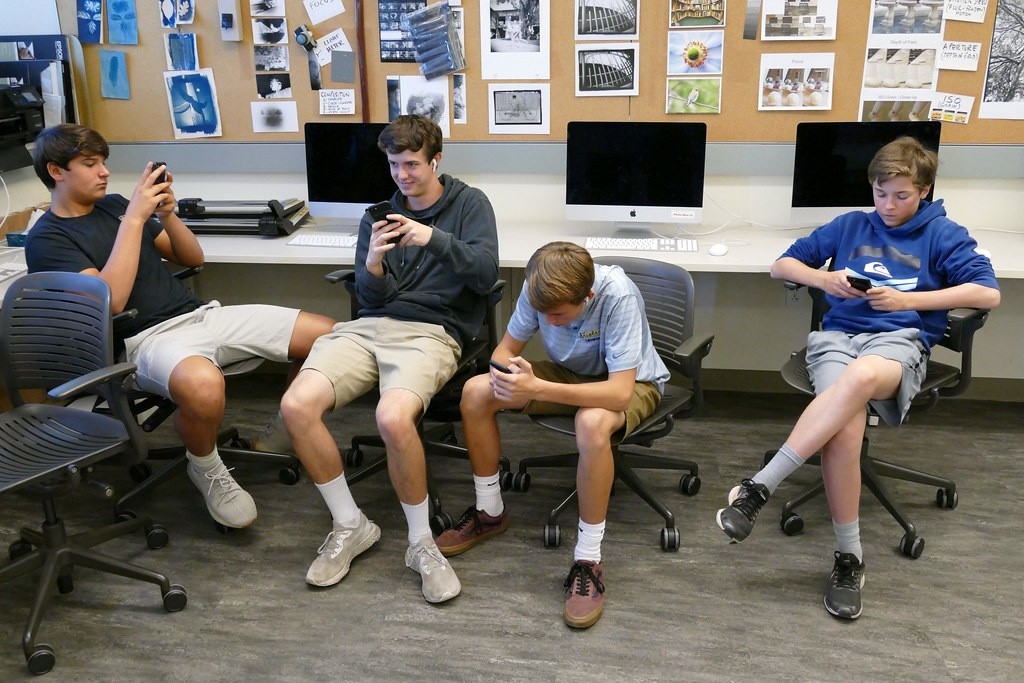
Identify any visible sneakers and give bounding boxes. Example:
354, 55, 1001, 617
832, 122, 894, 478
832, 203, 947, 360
563, 560, 605, 628
251, 410, 300, 458
186, 462, 257, 528
405, 536, 461, 603
715, 478, 770, 544
434, 501, 509, 555
824, 550, 866, 619
305, 510, 381, 586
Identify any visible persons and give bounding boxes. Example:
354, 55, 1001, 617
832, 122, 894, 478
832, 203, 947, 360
505, 20, 529, 48
434, 242, 670, 628
23, 123, 338, 530
511, 95, 519, 112
280, 115, 499, 604
717, 138, 1002, 618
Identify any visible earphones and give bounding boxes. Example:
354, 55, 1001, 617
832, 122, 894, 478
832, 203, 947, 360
431, 159, 437, 173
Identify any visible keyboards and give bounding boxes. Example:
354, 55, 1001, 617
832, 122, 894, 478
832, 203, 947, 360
585, 236, 698, 252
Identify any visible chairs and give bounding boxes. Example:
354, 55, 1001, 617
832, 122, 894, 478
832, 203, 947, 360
323, 267, 512, 535
0, 271, 189, 677
510, 254, 714, 554
62, 264, 307, 535
760, 278, 994, 560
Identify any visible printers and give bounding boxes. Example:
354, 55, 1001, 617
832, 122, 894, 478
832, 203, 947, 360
0, 83, 45, 145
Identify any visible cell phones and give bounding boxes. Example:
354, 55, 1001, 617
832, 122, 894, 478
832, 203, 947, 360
489, 360, 512, 375
287, 235, 358, 249
152, 161, 167, 207
846, 275, 872, 293
368, 200, 405, 244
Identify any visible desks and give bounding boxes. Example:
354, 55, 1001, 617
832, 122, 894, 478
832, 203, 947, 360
0, 174, 1024, 306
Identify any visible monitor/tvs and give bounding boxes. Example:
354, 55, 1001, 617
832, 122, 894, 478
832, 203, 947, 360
566, 121, 707, 239
790, 121, 941, 228
304, 123, 399, 220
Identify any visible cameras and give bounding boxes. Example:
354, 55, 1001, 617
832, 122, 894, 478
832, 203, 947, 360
293, 24, 317, 52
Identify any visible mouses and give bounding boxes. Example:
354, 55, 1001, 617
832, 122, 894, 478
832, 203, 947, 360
709, 243, 727, 256
